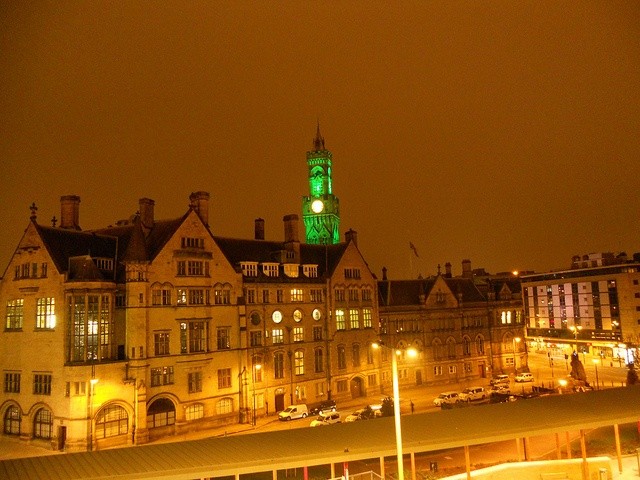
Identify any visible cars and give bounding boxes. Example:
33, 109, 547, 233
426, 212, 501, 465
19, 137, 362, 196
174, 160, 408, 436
345, 408, 367, 423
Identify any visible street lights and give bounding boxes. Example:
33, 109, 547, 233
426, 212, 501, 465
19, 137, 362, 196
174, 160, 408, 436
601, 351, 603, 366
253, 364, 261, 426
570, 326, 582, 354
513, 337, 520, 375
89, 379, 99, 451
371, 341, 418, 480
584, 350, 586, 364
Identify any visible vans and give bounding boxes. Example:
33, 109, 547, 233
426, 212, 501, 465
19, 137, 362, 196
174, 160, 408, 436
515, 373, 534, 382
459, 387, 488, 402
310, 412, 341, 427
433, 392, 459, 406
490, 375, 510, 385
487, 385, 510, 395
279, 404, 308, 421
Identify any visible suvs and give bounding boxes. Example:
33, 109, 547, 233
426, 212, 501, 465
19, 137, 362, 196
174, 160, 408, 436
310, 400, 336, 414
